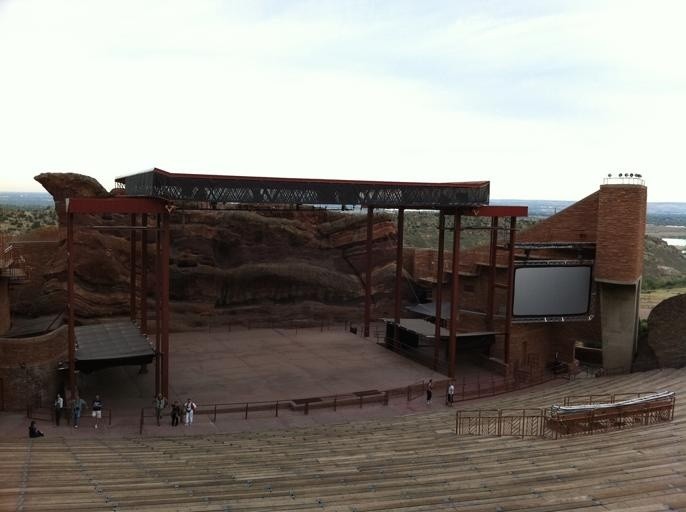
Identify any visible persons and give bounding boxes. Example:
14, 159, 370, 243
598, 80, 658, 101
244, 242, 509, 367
170, 401, 181, 427
54, 393, 63, 427
151, 393, 167, 427
183, 399, 198, 427
68, 395, 90, 429
446, 382, 457, 407
426, 379, 435, 405
90, 393, 104, 431
28, 420, 44, 438
